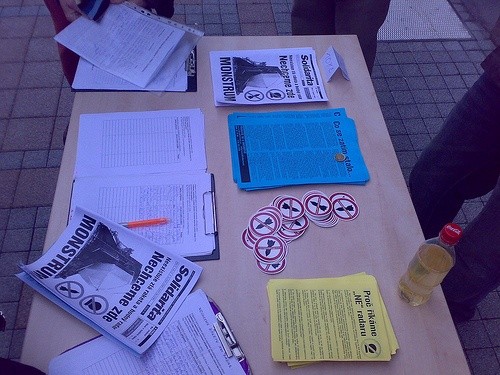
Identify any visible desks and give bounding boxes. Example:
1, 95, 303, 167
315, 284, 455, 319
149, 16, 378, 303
20, 34, 472, 375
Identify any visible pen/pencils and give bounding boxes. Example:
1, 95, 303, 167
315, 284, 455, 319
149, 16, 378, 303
118, 218, 170, 228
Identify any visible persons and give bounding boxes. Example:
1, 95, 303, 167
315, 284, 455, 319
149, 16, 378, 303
44, 0, 175, 86
0, 310, 49, 375
290, 0, 391, 76
409, 44, 500, 322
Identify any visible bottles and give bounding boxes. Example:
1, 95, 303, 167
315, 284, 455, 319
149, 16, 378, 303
398, 222, 463, 308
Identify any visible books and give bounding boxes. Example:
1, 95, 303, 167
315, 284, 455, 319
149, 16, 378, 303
15, 205, 204, 359
